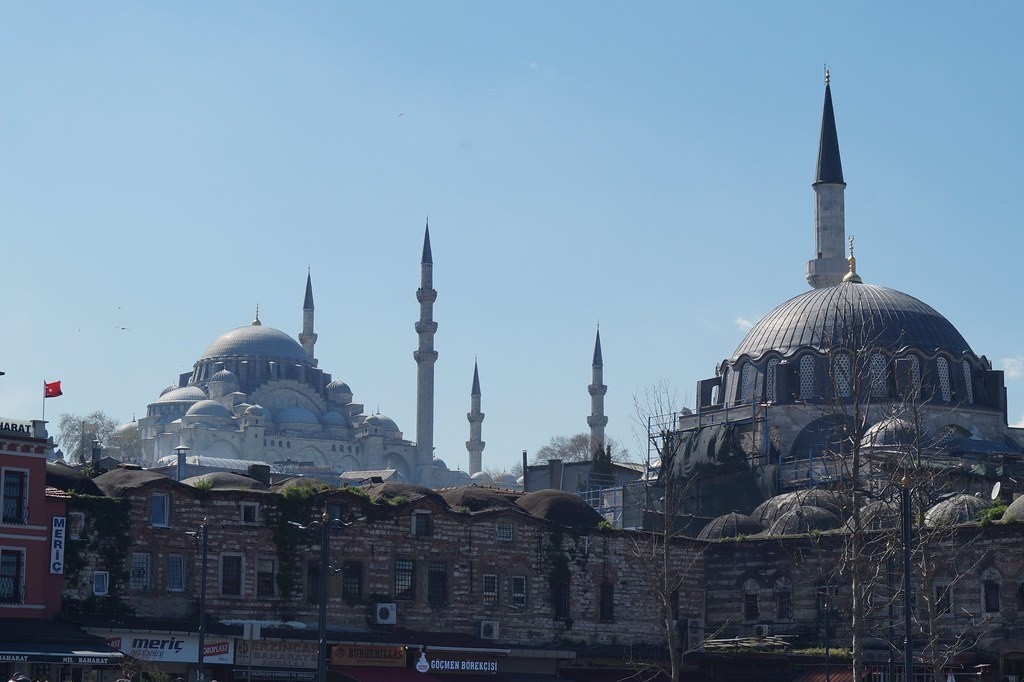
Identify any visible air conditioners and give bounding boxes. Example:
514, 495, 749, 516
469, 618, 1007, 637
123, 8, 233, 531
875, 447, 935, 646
372, 602, 397, 624
683, 618, 704, 651
477, 619, 499, 641
753, 624, 771, 637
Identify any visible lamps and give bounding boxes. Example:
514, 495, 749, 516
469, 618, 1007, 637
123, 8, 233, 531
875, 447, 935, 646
402, 643, 408, 649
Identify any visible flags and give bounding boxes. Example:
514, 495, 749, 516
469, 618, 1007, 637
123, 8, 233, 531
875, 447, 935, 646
45, 381, 63, 398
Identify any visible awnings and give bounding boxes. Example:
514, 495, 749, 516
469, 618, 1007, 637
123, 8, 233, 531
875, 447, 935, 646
560, 669, 716, 682
793, 667, 869, 682
337, 669, 442, 682
0, 641, 124, 665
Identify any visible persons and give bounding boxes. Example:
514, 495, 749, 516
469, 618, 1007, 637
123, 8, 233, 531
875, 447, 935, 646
8, 672, 30, 682
